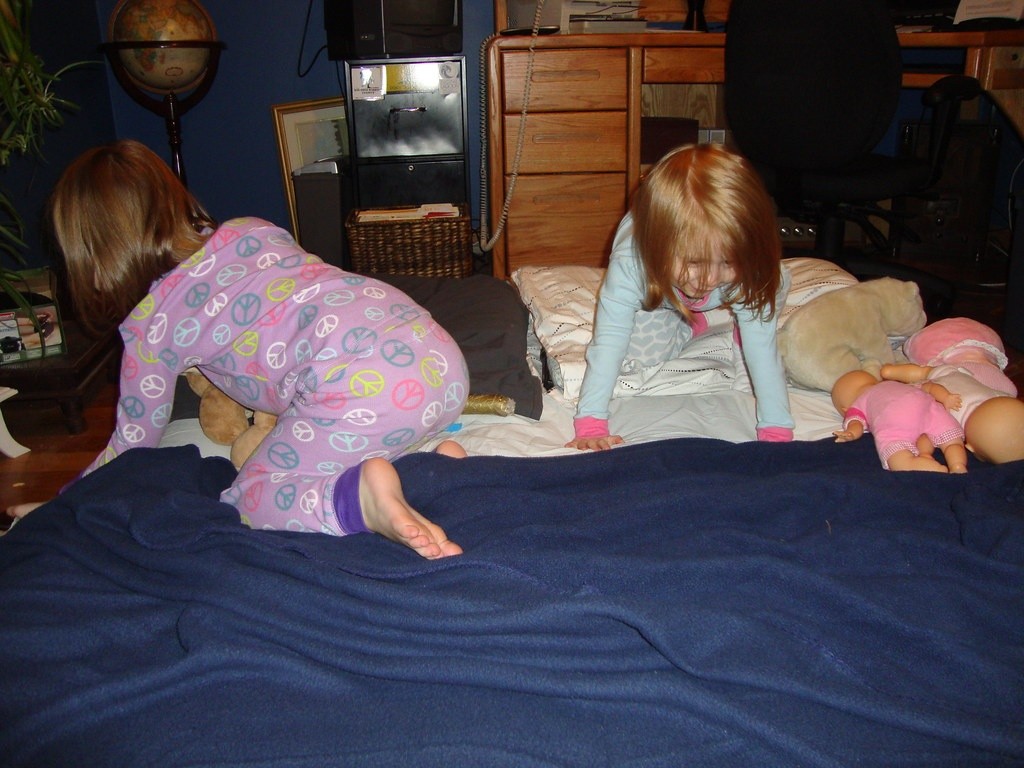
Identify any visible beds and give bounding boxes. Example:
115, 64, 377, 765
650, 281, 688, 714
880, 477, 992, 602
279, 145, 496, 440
0, 251, 1019, 768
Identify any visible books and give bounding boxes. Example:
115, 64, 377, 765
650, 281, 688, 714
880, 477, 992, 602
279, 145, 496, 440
355, 203, 460, 222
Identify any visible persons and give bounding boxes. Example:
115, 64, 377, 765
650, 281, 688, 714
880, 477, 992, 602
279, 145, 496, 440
831, 369, 969, 474
0, 139, 472, 563
563, 142, 799, 453
880, 363, 1024, 465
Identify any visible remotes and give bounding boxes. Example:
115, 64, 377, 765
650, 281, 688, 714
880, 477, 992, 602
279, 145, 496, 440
499, 25, 560, 36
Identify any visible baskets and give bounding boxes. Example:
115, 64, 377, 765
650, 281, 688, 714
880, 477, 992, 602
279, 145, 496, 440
345, 206, 472, 277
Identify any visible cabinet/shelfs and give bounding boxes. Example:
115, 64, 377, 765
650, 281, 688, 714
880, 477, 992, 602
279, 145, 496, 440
484, 22, 1024, 271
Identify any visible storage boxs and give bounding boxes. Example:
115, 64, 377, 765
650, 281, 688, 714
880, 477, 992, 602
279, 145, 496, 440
346, 201, 475, 279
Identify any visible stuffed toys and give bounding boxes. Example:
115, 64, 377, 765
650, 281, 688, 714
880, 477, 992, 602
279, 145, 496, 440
902, 316, 1018, 398
185, 371, 279, 473
775, 277, 927, 394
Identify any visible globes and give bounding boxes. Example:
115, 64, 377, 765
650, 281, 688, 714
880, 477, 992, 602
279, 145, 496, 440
97, 0, 228, 192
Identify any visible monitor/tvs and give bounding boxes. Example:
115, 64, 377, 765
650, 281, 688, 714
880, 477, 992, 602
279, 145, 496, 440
324, 0, 462, 60
